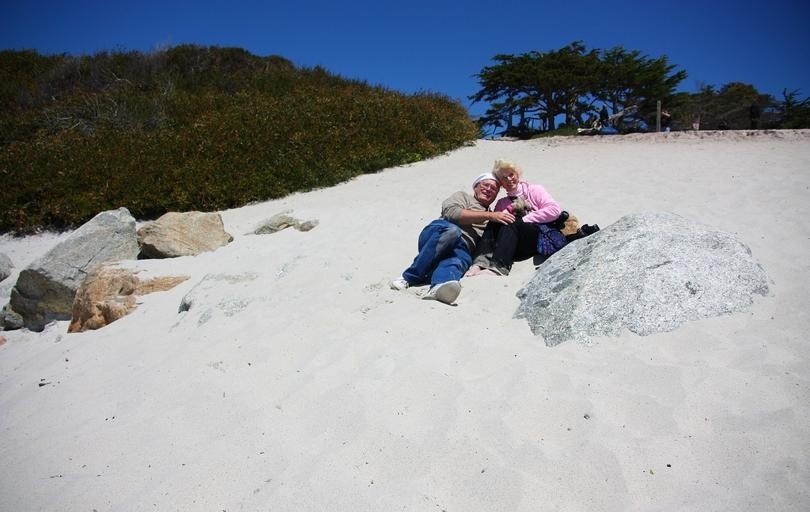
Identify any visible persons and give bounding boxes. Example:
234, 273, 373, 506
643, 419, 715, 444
600, 104, 610, 128
662, 108, 673, 132
391, 173, 516, 306
488, 155, 562, 278
692, 111, 701, 130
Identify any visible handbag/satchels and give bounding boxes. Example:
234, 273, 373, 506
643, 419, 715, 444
535, 223, 568, 257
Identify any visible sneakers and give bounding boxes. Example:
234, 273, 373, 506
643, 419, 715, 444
475, 254, 511, 276
565, 223, 600, 240
389, 279, 409, 290
422, 280, 461, 302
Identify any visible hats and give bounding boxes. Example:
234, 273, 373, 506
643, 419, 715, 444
471, 173, 502, 189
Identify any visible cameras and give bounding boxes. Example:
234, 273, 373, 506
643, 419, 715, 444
547, 211, 569, 228
